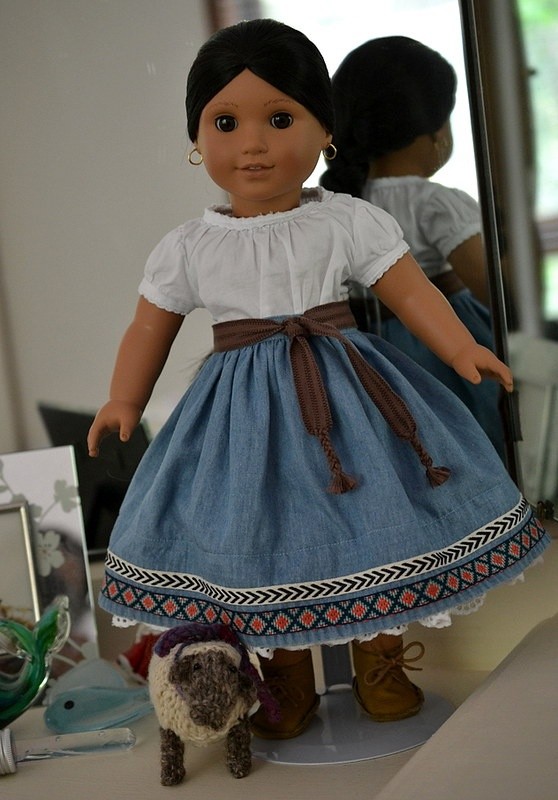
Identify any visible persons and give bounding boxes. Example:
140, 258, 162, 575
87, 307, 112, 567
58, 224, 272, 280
72, 16, 554, 741
314, 34, 519, 482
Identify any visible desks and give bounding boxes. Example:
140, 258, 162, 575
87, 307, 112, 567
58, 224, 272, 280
0, 649, 498, 800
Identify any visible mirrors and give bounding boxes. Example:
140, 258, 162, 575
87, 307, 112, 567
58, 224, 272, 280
200, 0, 532, 501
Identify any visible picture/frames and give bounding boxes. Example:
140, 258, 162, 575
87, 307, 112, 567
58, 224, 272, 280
0, 501, 41, 631
